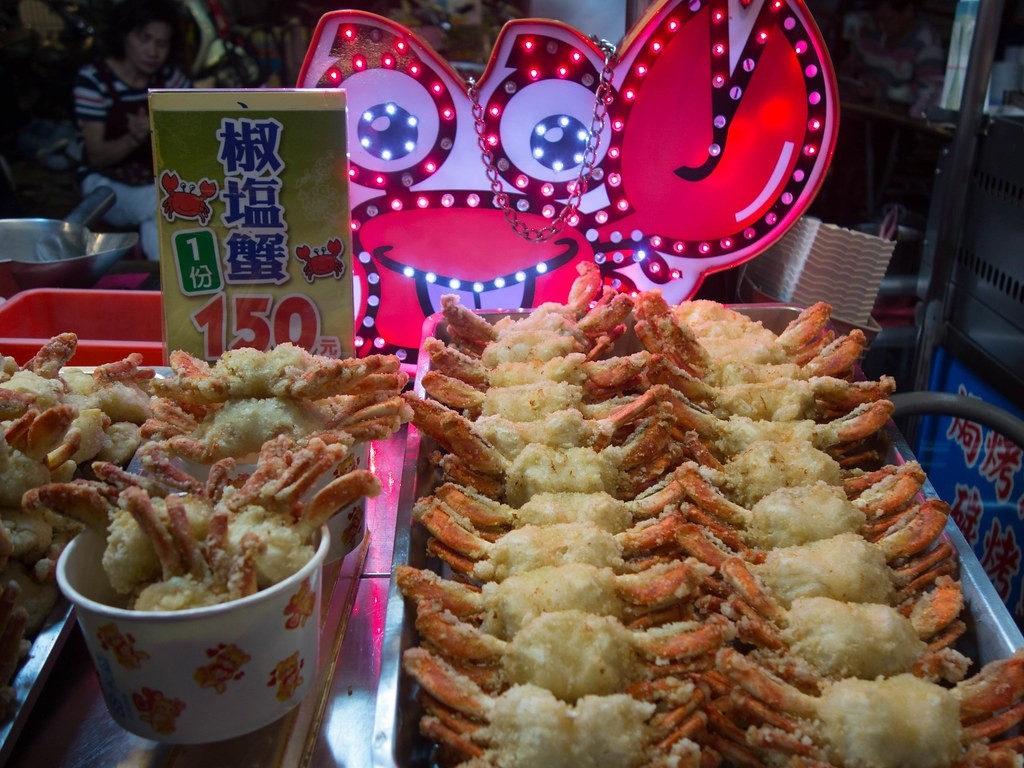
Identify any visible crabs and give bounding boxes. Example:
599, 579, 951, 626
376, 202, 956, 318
293, 1, 840, 376
0, 331, 410, 728
405, 260, 1024, 768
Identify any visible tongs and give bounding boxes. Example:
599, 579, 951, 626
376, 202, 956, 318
874, 204, 906, 241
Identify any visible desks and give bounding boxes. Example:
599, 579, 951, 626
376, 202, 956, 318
838, 91, 958, 228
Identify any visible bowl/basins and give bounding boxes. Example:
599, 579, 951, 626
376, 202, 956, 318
55, 380, 370, 743
849, 222, 924, 273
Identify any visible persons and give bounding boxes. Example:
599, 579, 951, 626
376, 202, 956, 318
839, 0, 943, 211
71, 0, 194, 261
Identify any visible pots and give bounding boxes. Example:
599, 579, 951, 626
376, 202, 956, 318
0, 186, 139, 300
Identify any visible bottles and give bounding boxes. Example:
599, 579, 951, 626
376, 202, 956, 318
223, 16, 308, 87
